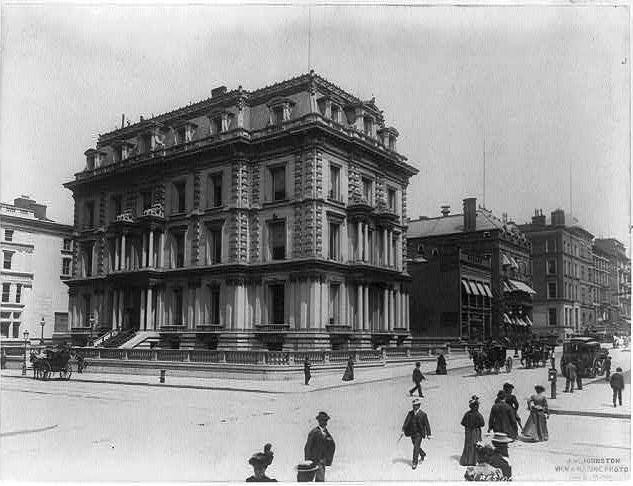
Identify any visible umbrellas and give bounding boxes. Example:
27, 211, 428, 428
412, 462, 417, 469
421, 453, 426, 460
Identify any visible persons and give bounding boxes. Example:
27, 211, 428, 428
338, 353, 356, 380
304, 412, 335, 482
435, 323, 613, 481
402, 399, 431, 470
409, 362, 427, 398
302, 357, 314, 387
75, 348, 84, 374
610, 367, 625, 407
294, 457, 317, 482
246, 443, 279, 482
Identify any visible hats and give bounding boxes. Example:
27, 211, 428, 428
560, 336, 608, 379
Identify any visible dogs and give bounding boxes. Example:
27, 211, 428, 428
468, 349, 490, 376
29, 352, 49, 380
522, 344, 540, 369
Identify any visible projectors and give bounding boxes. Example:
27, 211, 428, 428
517, 329, 559, 367
468, 336, 515, 375
30, 338, 73, 380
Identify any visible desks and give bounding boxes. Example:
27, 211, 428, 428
40, 317, 46, 345
21, 329, 29, 375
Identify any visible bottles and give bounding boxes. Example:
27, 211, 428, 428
536, 384, 545, 393
411, 399, 421, 405
315, 411, 331, 421
